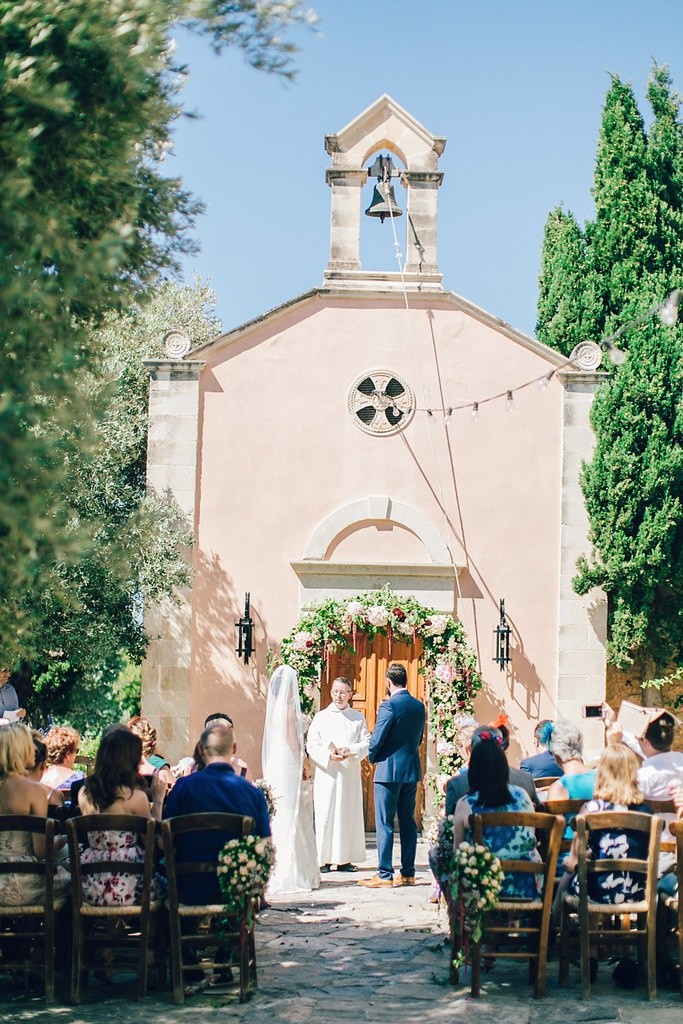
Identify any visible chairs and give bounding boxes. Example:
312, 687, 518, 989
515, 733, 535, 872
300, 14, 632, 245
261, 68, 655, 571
0, 756, 258, 1009
444, 776, 683, 1001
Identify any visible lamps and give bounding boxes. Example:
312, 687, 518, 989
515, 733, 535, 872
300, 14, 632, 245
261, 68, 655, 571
234, 592, 256, 666
492, 599, 512, 673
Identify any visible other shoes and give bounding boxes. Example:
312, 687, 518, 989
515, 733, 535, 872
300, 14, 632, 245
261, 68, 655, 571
589, 955, 599, 980
210, 968, 233, 986
337, 863, 358, 872
191, 969, 207, 986
320, 865, 332, 873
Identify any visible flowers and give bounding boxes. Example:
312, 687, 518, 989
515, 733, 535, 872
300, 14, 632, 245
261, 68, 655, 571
266, 582, 482, 850
447, 841, 505, 970
252, 777, 287, 824
212, 834, 277, 931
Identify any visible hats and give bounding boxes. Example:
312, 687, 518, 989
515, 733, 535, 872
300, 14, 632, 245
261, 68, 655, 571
645, 712, 675, 751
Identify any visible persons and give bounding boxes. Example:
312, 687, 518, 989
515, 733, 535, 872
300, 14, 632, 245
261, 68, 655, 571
0, 713, 272, 994
0, 668, 26, 722
428, 701, 683, 986
305, 677, 371, 873
358, 663, 425, 888
261, 666, 320, 893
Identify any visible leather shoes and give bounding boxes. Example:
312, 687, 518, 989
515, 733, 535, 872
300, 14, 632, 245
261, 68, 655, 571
397, 874, 416, 885
357, 874, 394, 888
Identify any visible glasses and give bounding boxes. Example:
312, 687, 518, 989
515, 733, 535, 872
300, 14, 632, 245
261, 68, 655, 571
3, 668, 11, 673
73, 749, 79, 754
332, 690, 347, 697
